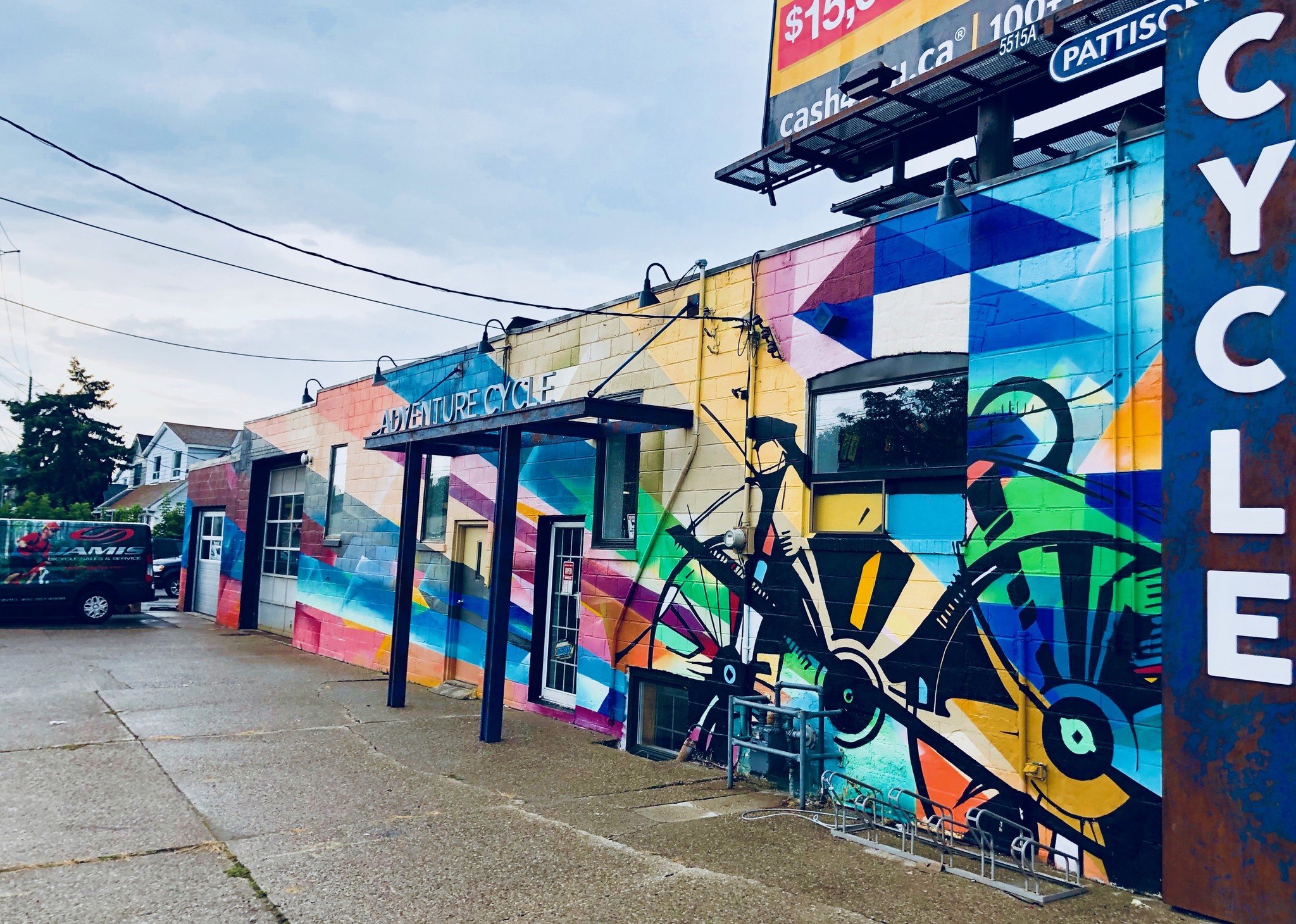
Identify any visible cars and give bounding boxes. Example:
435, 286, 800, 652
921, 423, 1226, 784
151, 536, 225, 599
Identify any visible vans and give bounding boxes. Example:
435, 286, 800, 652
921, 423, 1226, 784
0, 519, 159, 626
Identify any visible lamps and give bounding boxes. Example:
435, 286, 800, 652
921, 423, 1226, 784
478, 318, 506, 355
838, 60, 903, 100
637, 263, 673, 309
936, 158, 977, 222
301, 379, 325, 405
371, 356, 399, 388
